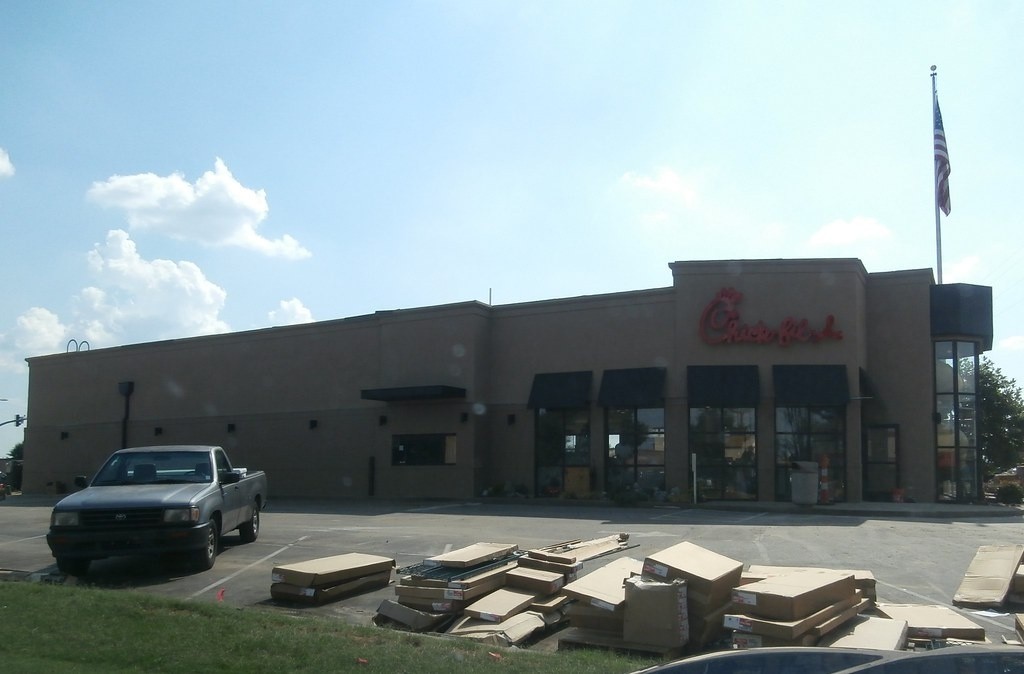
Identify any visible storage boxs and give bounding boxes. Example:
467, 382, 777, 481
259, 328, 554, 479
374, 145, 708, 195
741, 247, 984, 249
269, 531, 1023, 653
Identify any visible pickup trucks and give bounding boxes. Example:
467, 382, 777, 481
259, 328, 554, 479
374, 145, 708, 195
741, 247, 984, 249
46, 445, 267, 574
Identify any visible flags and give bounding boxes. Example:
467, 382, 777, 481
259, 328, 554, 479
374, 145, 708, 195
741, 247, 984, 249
931, 78, 953, 217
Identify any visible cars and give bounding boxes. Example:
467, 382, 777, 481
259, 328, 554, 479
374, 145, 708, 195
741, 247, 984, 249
0, 471, 8, 499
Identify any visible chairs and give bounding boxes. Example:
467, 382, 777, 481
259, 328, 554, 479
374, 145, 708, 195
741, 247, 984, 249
135, 464, 156, 481
195, 463, 209, 476
